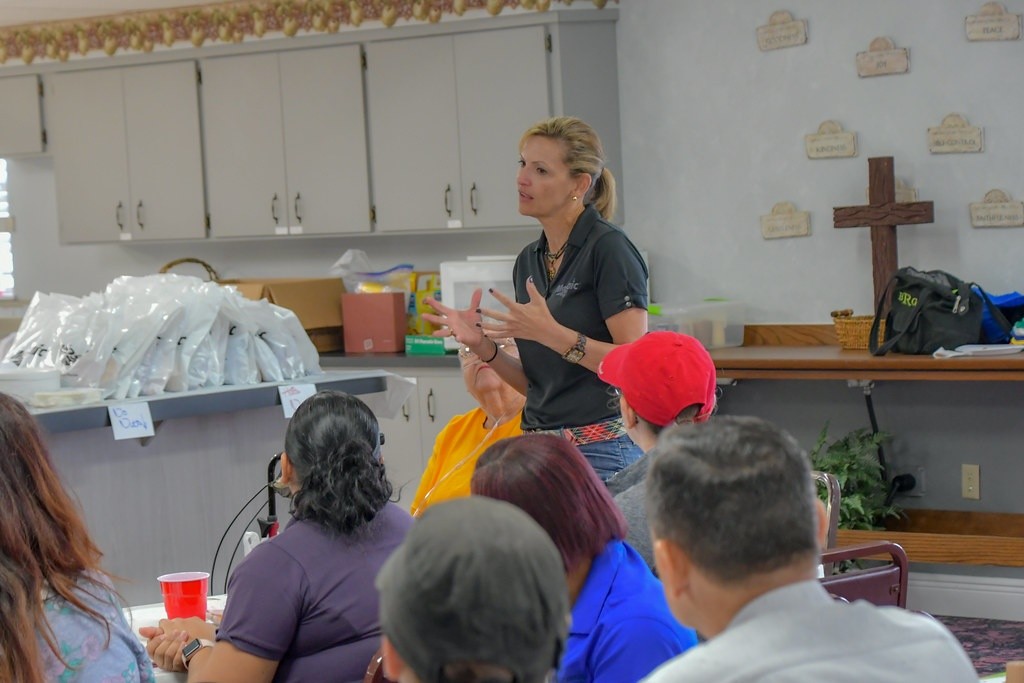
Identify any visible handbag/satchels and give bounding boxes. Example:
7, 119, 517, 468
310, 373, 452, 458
868, 266, 1024, 361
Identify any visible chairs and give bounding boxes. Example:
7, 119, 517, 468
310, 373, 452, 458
818, 539, 907, 608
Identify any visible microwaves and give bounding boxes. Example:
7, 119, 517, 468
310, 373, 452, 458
437, 252, 649, 350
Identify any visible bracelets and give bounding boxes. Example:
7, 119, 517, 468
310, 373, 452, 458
482, 341, 498, 363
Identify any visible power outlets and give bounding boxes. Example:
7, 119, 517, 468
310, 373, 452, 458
897, 467, 925, 496
962, 463, 980, 500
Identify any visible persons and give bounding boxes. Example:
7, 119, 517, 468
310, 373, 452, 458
375, 496, 570, 682
410, 330, 526, 517
0, 393, 156, 683
421, 117, 650, 478
597, 330, 718, 576
641, 417, 980, 683
138, 391, 415, 683
469, 434, 698, 683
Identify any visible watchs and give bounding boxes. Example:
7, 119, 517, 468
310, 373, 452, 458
181, 637, 214, 669
562, 332, 586, 363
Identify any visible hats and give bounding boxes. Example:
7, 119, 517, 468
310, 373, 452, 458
598, 331, 716, 427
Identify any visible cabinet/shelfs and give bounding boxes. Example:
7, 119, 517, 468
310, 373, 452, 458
365, 7, 624, 238
42, 46, 202, 248
0, 76, 50, 167
200, 29, 371, 243
374, 369, 481, 516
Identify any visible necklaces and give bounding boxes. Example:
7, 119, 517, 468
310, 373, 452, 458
545, 240, 567, 280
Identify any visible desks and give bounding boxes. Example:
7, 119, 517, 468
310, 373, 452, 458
700, 345, 1024, 569
123, 594, 228, 683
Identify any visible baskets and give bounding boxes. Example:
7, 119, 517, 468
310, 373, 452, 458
834, 311, 887, 350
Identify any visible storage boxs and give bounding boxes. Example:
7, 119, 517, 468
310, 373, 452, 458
404, 334, 455, 357
340, 293, 409, 353
216, 278, 346, 351
647, 303, 744, 349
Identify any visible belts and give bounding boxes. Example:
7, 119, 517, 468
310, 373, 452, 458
523, 420, 626, 446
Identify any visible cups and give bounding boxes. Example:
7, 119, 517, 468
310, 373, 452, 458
158, 572, 210, 621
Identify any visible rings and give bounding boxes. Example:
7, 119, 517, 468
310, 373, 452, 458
450, 329, 452, 335
